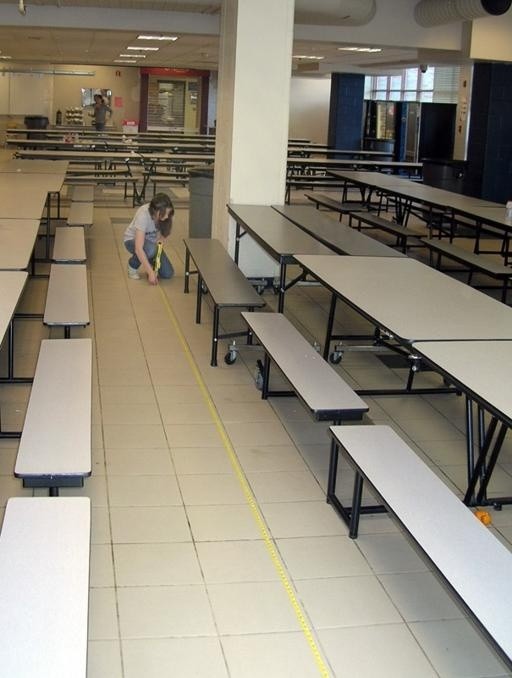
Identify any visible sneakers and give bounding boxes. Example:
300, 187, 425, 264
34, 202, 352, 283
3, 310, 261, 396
128, 262, 140, 280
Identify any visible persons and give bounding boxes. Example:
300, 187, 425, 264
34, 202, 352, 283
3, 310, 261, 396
87, 94, 113, 131
122, 193, 175, 286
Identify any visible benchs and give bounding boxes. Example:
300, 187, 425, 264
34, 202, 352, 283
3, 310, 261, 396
305, 190, 361, 222
184, 236, 265, 375
68, 187, 93, 227
41, 262, 91, 338
48, 134, 216, 208
286, 175, 362, 202
1, 494, 91, 675
323, 421, 512, 672
238, 308, 371, 420
52, 226, 88, 263
12, 336, 94, 496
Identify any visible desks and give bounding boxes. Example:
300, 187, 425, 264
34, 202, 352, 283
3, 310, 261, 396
227, 204, 511, 512
1, 160, 68, 346
7, 128, 216, 162
0, 187, 49, 345
288, 136, 425, 170
327, 170, 509, 244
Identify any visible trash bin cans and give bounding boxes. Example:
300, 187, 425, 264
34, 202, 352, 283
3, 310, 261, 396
363, 137, 396, 174
187, 167, 214, 239
24, 117, 48, 139
420, 158, 477, 222
